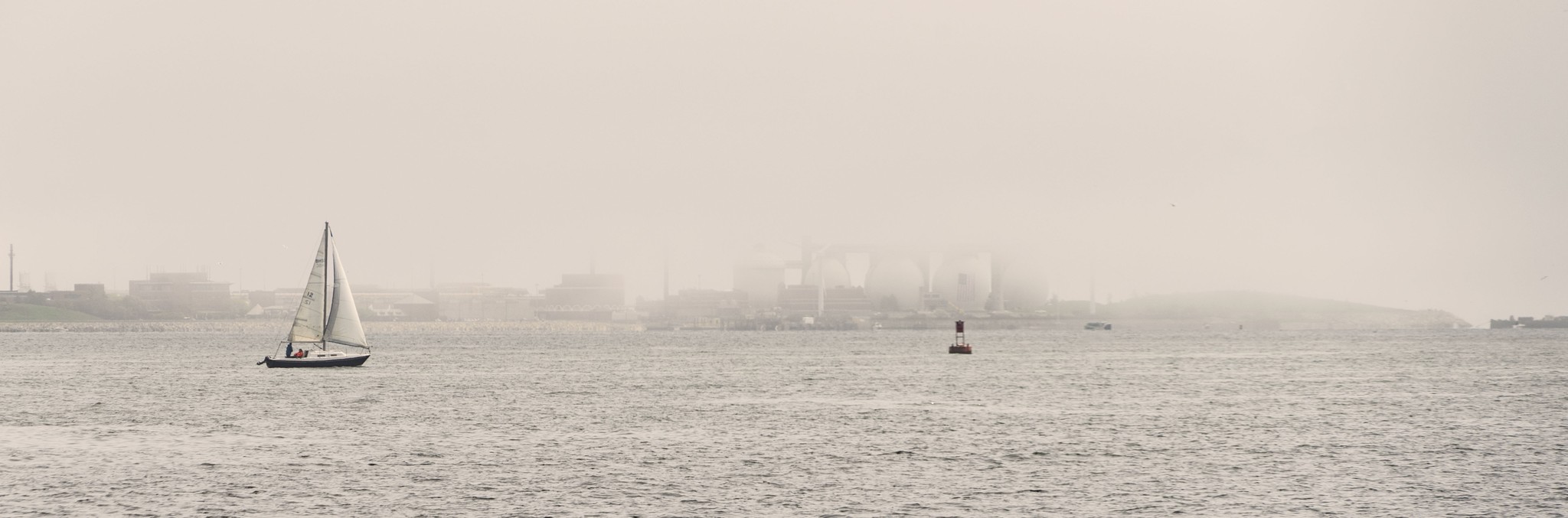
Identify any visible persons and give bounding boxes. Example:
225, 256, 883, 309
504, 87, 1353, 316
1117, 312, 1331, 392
285, 349, 303, 358
286, 343, 293, 358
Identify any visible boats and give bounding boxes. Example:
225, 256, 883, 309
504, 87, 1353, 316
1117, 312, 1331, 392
1083, 321, 1112, 330
948, 320, 972, 355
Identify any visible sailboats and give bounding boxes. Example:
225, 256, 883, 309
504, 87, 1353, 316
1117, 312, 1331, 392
256, 220, 373, 368
